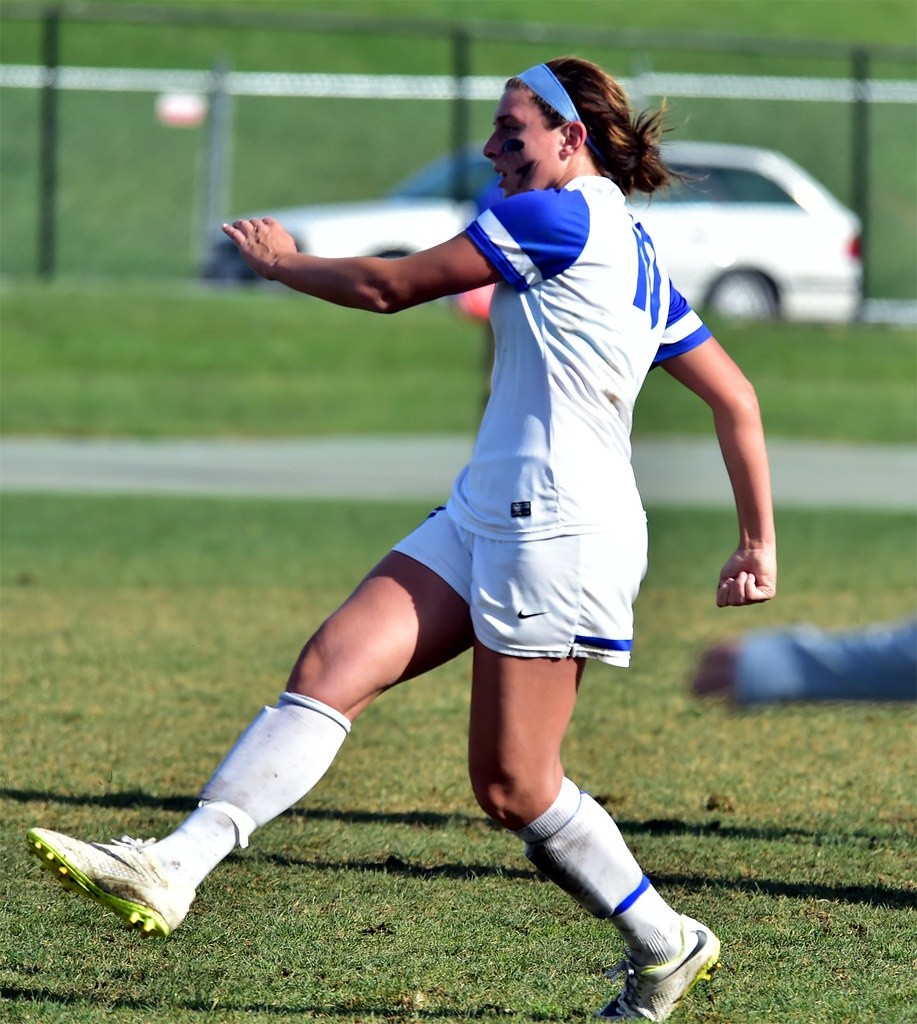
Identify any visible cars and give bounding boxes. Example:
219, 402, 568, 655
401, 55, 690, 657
198, 138, 866, 321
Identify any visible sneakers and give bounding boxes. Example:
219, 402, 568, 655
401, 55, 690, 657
25, 826, 197, 943
598, 912, 723, 1023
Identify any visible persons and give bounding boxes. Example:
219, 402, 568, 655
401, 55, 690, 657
688, 622, 914, 709
27, 57, 783, 1023
